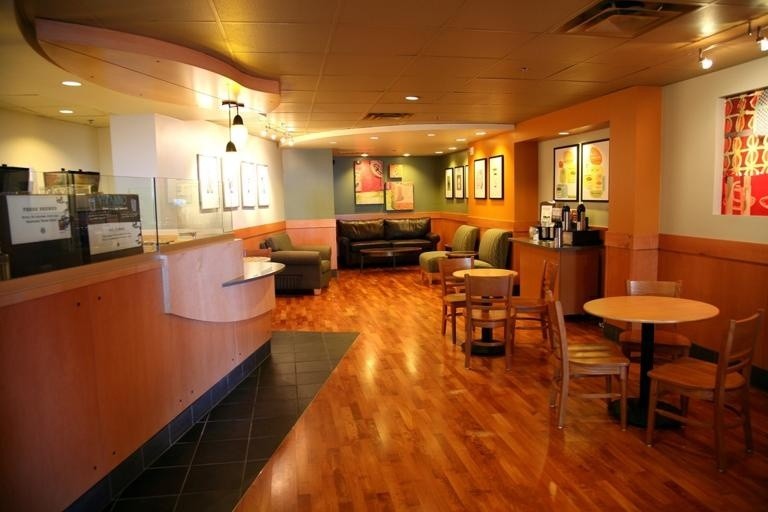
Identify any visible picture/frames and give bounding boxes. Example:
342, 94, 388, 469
475, 158, 487, 199
444, 165, 468, 199
489, 155, 504, 198
553, 143, 579, 202
581, 138, 609, 202
197, 155, 270, 210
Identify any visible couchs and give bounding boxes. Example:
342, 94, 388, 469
336, 218, 441, 268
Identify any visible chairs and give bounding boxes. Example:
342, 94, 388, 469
260, 233, 332, 296
464, 273, 515, 372
473, 228, 512, 268
620, 279, 691, 429
543, 301, 629, 431
513, 255, 560, 353
419, 224, 478, 285
244, 247, 272, 265
646, 308, 763, 470
437, 255, 475, 344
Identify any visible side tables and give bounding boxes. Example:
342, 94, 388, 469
241, 255, 271, 263
445, 251, 478, 259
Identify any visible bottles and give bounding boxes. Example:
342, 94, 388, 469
561, 206, 570, 231
577, 204, 586, 231
553, 221, 563, 248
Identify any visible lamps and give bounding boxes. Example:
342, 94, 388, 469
222, 100, 244, 153
699, 25, 768, 70
